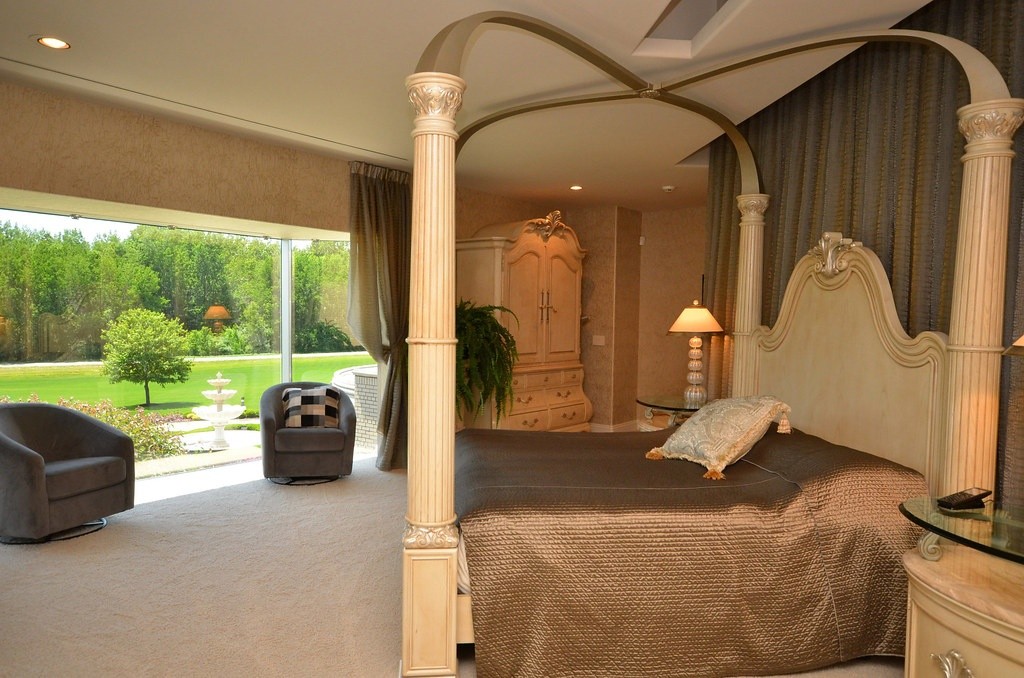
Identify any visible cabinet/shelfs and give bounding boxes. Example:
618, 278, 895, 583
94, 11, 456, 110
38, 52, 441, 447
454, 209, 593, 433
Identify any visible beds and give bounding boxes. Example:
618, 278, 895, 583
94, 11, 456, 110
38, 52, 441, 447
399, 10, 1024, 676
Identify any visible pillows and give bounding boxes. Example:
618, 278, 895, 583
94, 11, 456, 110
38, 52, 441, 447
281, 386, 340, 429
646, 394, 793, 479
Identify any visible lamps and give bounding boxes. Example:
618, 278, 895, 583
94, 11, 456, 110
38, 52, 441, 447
203, 305, 232, 334
665, 299, 724, 398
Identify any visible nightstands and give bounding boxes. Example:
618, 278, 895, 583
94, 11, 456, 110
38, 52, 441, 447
898, 495, 1024, 677
636, 394, 706, 431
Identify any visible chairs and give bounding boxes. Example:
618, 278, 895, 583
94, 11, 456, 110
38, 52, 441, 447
259, 381, 356, 484
0, 401, 135, 543
